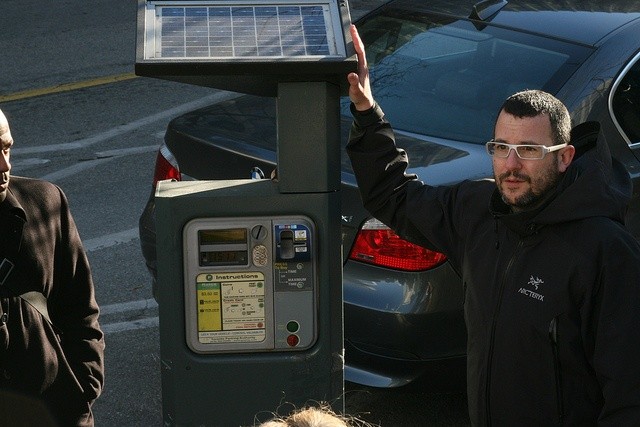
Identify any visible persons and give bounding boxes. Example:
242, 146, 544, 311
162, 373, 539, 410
0, 109, 105, 427
347, 25, 640, 427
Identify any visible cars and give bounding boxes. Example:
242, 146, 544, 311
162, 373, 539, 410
136, 2, 638, 426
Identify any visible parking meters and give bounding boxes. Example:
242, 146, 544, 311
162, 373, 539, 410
134, 5, 357, 422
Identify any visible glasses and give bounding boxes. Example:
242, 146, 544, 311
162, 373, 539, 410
486, 139, 568, 161
